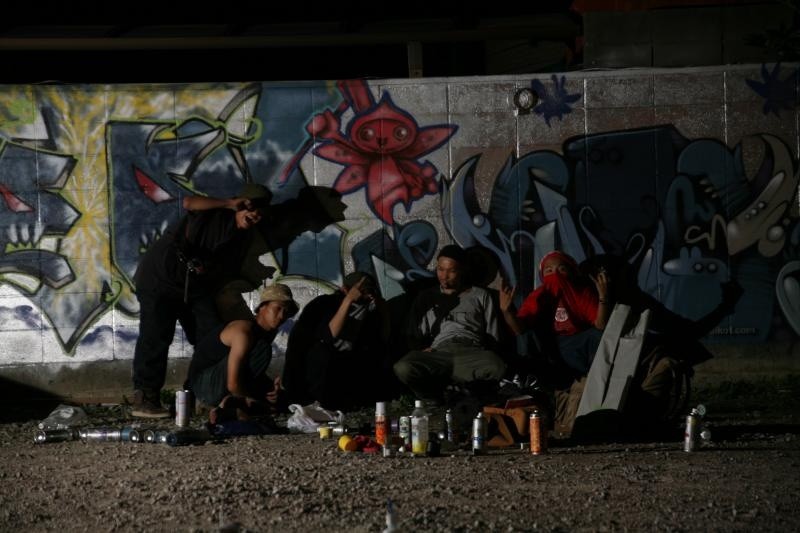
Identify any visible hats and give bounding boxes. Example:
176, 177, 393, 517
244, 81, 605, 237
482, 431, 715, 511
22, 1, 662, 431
254, 284, 300, 318
538, 250, 575, 282
242, 184, 274, 208
436, 245, 466, 263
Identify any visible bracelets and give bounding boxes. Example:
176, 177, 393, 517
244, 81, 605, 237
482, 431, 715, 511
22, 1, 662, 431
598, 296, 609, 304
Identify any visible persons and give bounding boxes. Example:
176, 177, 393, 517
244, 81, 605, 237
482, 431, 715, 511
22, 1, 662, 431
280, 271, 378, 413
128, 185, 272, 419
190, 284, 301, 425
392, 245, 509, 407
498, 250, 612, 395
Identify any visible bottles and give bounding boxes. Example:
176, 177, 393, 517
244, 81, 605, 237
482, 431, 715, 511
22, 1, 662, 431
446, 407, 459, 442
471, 412, 488, 454
529, 409, 548, 456
683, 408, 701, 452
374, 400, 442, 457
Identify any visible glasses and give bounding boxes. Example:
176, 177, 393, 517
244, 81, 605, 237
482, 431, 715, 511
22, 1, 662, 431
247, 206, 263, 216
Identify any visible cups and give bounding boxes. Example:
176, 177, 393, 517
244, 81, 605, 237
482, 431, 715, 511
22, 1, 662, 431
319, 427, 334, 439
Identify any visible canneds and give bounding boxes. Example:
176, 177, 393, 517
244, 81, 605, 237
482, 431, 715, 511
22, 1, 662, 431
175, 390, 191, 428
34, 427, 169, 443
398, 415, 412, 444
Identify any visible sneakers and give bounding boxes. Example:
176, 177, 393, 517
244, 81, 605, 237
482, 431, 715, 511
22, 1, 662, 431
131, 389, 170, 419
304, 402, 335, 422
